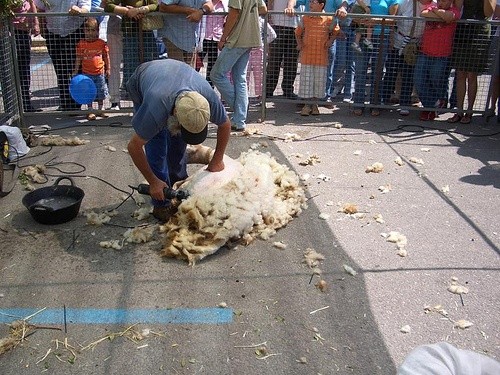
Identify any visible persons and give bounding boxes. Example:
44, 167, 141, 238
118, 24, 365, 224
35, 0, 91, 115
265, 0, 500, 124
9, 0, 43, 112
210, 0, 268, 131
90, 0, 229, 110
246, 15, 278, 106
72, 16, 111, 120
125, 59, 231, 222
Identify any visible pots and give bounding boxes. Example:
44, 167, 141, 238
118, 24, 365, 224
22, 176, 85, 226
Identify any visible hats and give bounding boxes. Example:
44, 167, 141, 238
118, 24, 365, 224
176, 91, 210, 145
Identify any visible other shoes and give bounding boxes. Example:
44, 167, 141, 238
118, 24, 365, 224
401, 109, 409, 115
87, 113, 96, 120
302, 106, 318, 116
371, 110, 379, 116
264, 93, 273, 98
106, 105, 122, 110
351, 42, 362, 53
448, 113, 472, 124
97, 110, 109, 118
419, 111, 435, 122
320, 91, 355, 103
153, 207, 172, 222
230, 124, 244, 131
284, 92, 297, 100
411, 96, 421, 104
363, 38, 373, 49
353, 107, 363, 116
387, 96, 399, 104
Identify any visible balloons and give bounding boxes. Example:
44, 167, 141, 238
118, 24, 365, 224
69, 74, 97, 104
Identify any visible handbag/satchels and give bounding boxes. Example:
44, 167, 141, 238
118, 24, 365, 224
143, 16, 164, 30
403, 42, 420, 66
